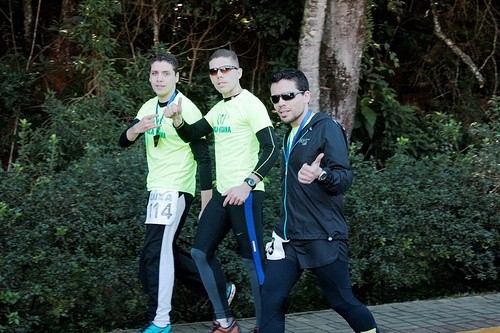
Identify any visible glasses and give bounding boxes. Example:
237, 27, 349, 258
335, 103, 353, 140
208, 65, 238, 76
270, 91, 304, 104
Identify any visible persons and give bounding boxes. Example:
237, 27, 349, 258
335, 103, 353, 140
117, 53, 238, 333
162, 47, 282, 333
261, 69, 378, 333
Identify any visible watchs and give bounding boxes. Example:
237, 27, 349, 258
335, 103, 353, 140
317, 169, 327, 181
244, 178, 256, 191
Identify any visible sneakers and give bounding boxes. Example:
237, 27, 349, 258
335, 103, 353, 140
136, 321, 173, 333
223, 282, 236, 304
208, 320, 241, 333
